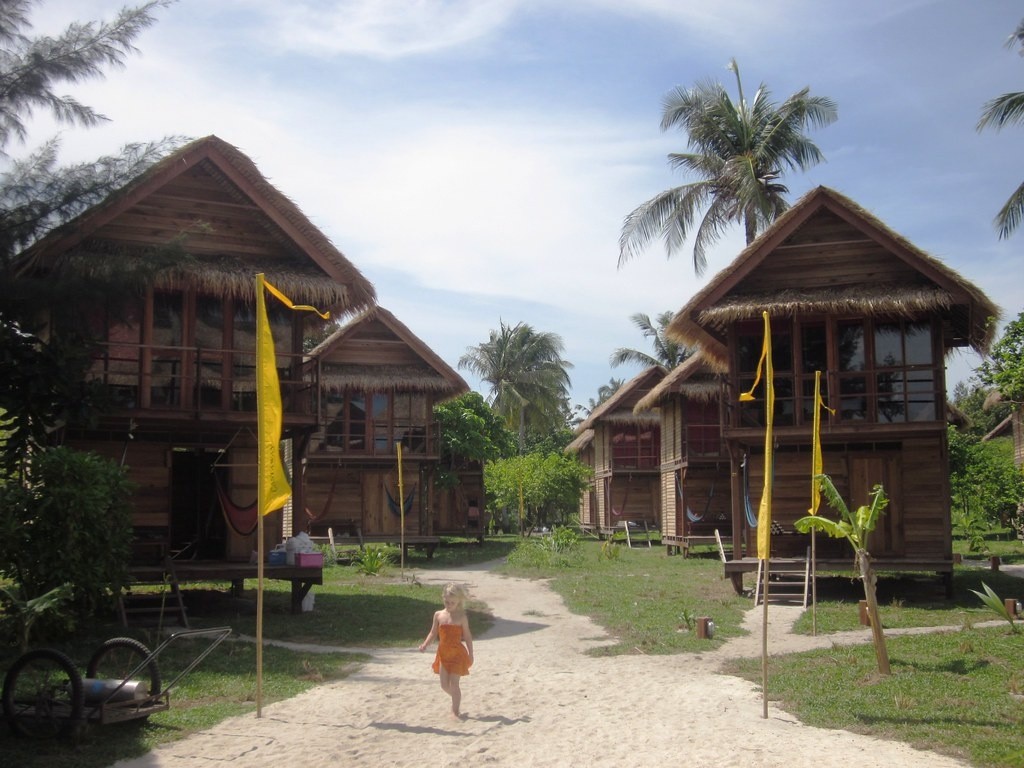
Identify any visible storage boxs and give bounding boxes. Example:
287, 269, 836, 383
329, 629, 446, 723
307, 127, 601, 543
294, 551, 324, 568
268, 550, 287, 566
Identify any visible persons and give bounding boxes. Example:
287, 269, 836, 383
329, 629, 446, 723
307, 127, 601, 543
418, 582, 474, 717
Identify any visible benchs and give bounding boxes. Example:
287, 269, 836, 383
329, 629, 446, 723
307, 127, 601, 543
687, 522, 732, 536
